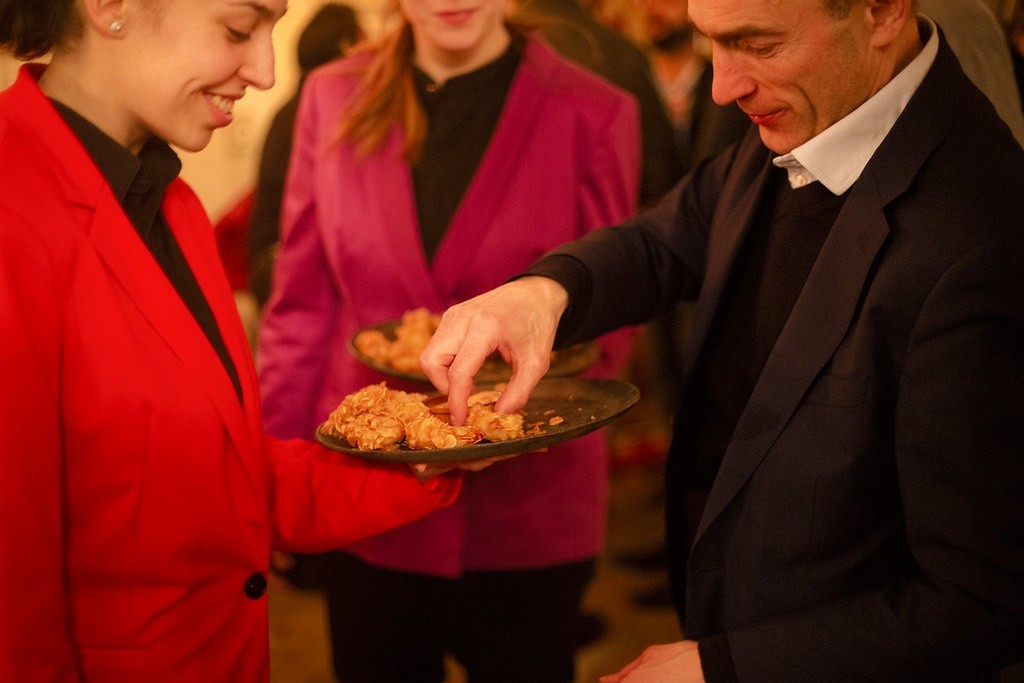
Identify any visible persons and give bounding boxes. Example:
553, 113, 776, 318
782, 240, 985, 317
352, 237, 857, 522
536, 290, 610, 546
255, 1, 643, 683
416, 0, 1022, 683
249, 0, 761, 317
0, 0, 545, 682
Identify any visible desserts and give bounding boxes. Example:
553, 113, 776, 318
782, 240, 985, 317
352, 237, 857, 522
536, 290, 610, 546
319, 308, 565, 451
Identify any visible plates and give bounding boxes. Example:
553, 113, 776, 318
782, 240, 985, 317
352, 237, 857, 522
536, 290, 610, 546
316, 377, 643, 465
349, 320, 600, 387
